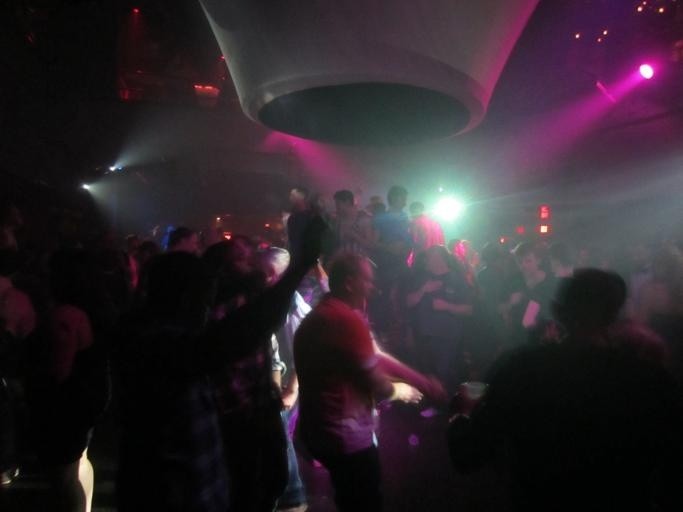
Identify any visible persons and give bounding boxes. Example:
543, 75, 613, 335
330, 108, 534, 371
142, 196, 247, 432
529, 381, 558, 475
0, 180, 683, 511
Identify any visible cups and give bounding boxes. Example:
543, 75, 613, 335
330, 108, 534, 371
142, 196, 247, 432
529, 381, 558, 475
460, 382, 485, 416
